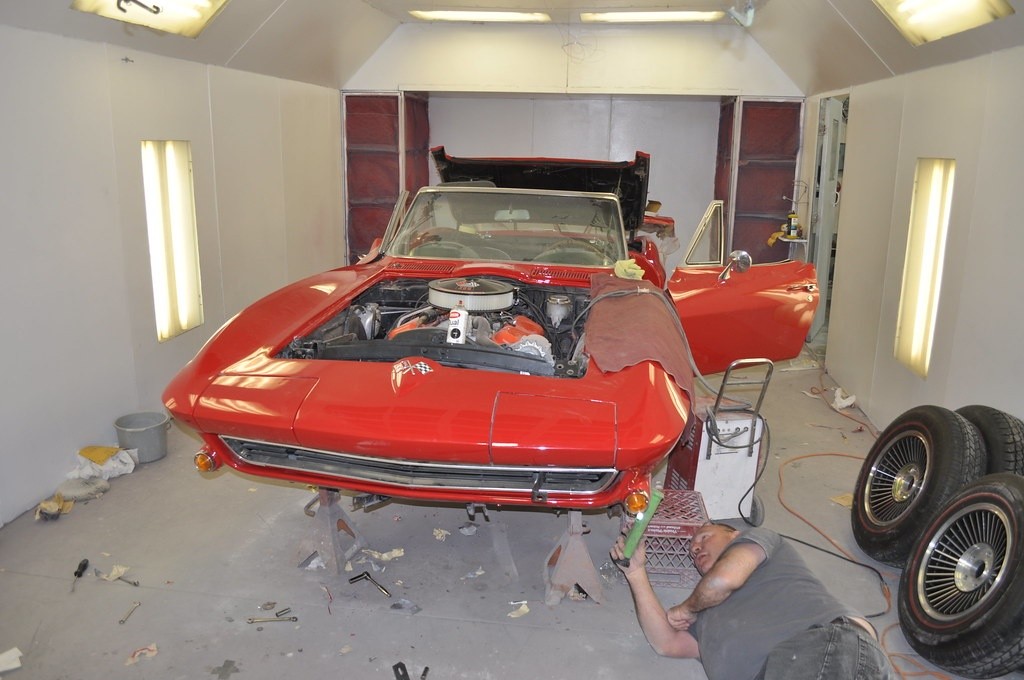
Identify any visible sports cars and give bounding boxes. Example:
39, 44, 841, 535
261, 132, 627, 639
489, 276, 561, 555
160, 140, 822, 516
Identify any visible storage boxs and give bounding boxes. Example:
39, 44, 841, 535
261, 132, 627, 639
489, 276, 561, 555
619, 491, 711, 589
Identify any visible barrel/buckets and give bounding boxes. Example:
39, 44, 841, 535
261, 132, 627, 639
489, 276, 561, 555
114, 411, 171, 464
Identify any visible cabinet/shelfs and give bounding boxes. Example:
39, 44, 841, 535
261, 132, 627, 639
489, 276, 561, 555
708, 95, 806, 265
341, 89, 429, 264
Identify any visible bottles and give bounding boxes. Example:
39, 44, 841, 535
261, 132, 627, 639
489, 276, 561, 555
787, 211, 798, 239
446, 300, 469, 345
192, 442, 223, 474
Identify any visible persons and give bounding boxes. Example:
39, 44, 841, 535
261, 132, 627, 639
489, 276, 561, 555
610, 524, 897, 680
